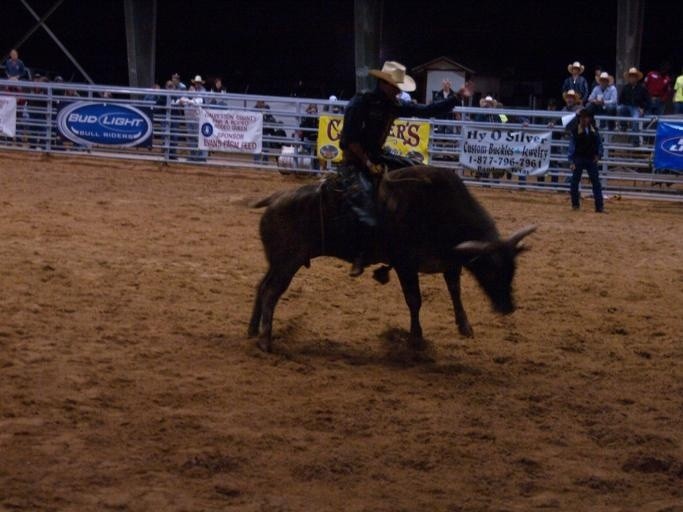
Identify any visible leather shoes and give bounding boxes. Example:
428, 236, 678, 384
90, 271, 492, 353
349, 257, 362, 276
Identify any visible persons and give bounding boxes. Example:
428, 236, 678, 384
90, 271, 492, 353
252, 95, 344, 179
337, 57, 477, 282
567, 107, 608, 214
537, 60, 683, 190
396, 78, 530, 190
1, 48, 115, 152
142, 72, 227, 156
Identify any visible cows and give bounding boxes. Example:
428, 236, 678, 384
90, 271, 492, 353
247, 164, 542, 354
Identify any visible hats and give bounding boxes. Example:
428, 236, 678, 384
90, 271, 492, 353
567, 61, 584, 74
367, 60, 416, 92
575, 108, 594, 117
594, 72, 613, 85
622, 66, 643, 80
562, 89, 579, 102
479, 96, 497, 108
191, 75, 206, 84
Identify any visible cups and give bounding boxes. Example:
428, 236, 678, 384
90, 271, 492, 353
597, 94, 603, 101
